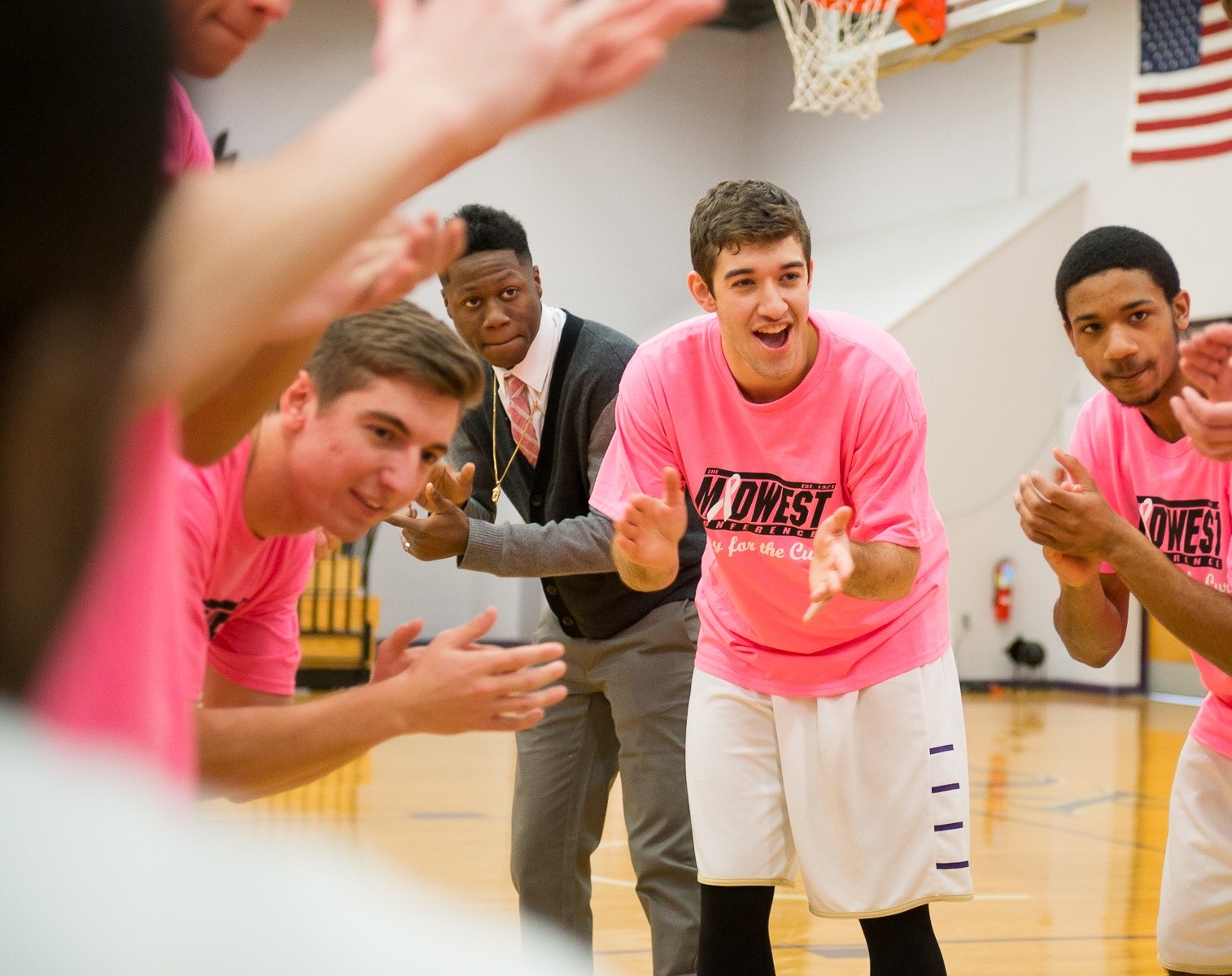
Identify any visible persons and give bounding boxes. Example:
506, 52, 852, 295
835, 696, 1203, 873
380, 204, 717, 976
0, 0, 725, 976
588, 180, 974, 976
1014, 227, 1232, 976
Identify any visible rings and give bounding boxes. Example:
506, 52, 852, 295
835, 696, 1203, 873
403, 541, 410, 552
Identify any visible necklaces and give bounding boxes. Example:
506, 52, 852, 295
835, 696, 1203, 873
491, 372, 541, 502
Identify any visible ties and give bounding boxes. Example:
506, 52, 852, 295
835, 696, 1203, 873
504, 375, 540, 467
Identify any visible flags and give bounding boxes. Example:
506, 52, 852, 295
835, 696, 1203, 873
1132, 0, 1232, 165
